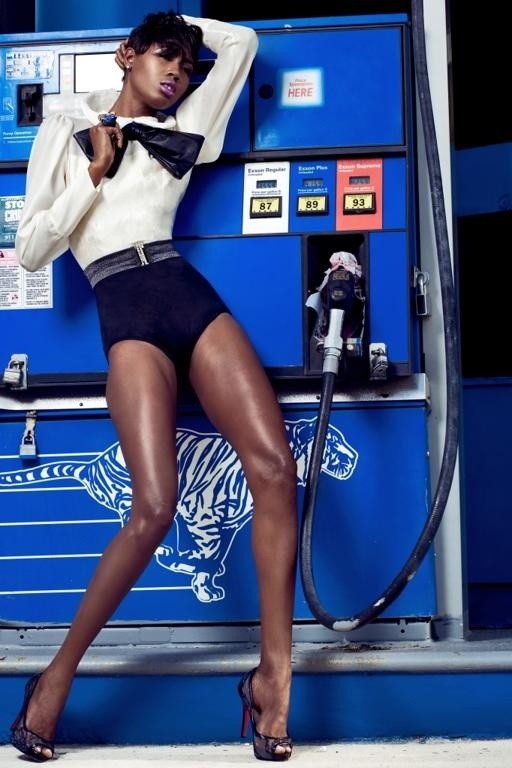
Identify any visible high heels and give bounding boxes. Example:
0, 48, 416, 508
237, 667, 292, 760
10, 672, 58, 762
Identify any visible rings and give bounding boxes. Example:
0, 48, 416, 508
97, 111, 118, 128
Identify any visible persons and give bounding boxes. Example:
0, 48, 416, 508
9, 7, 297, 763
